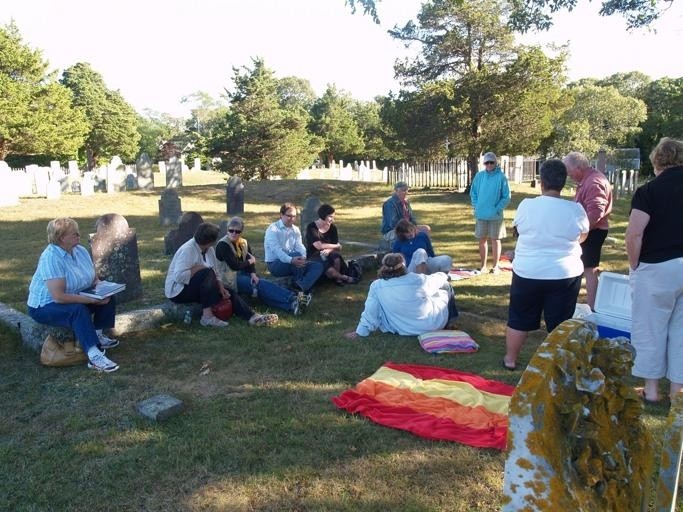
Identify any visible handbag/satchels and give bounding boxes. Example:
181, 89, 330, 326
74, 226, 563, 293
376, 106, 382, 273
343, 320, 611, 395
214, 298, 232, 320
40, 334, 88, 366
348, 259, 362, 278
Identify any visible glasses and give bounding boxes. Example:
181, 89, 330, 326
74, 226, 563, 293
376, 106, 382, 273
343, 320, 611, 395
286, 213, 296, 218
486, 161, 494, 165
228, 229, 241, 234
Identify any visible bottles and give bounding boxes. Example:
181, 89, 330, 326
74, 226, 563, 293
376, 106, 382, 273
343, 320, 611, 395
183, 310, 192, 324
250, 282, 258, 298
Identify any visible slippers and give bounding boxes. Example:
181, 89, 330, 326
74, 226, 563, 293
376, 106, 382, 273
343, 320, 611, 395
635, 387, 656, 406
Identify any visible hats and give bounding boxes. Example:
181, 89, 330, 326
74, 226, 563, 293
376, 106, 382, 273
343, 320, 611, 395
394, 182, 411, 191
483, 152, 497, 163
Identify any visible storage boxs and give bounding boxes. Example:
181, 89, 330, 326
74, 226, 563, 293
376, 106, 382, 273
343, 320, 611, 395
582, 272, 631, 339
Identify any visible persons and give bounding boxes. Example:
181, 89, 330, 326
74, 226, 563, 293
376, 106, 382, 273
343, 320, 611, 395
263, 203, 324, 302
502, 158, 590, 370
344, 181, 459, 341
626, 135, 683, 402
561, 152, 613, 314
214, 216, 312, 316
164, 223, 279, 329
305, 203, 359, 287
27, 218, 121, 374
469, 152, 511, 275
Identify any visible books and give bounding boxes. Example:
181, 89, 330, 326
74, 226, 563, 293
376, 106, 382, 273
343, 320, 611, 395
79, 280, 127, 300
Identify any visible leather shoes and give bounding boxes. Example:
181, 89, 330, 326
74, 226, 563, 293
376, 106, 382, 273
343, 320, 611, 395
335, 280, 344, 287
349, 277, 359, 284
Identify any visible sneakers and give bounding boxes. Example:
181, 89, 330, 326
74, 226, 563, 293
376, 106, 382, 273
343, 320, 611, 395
490, 268, 499, 275
88, 351, 119, 372
288, 283, 315, 316
248, 314, 278, 327
98, 334, 119, 348
199, 315, 228, 327
475, 269, 486, 274
